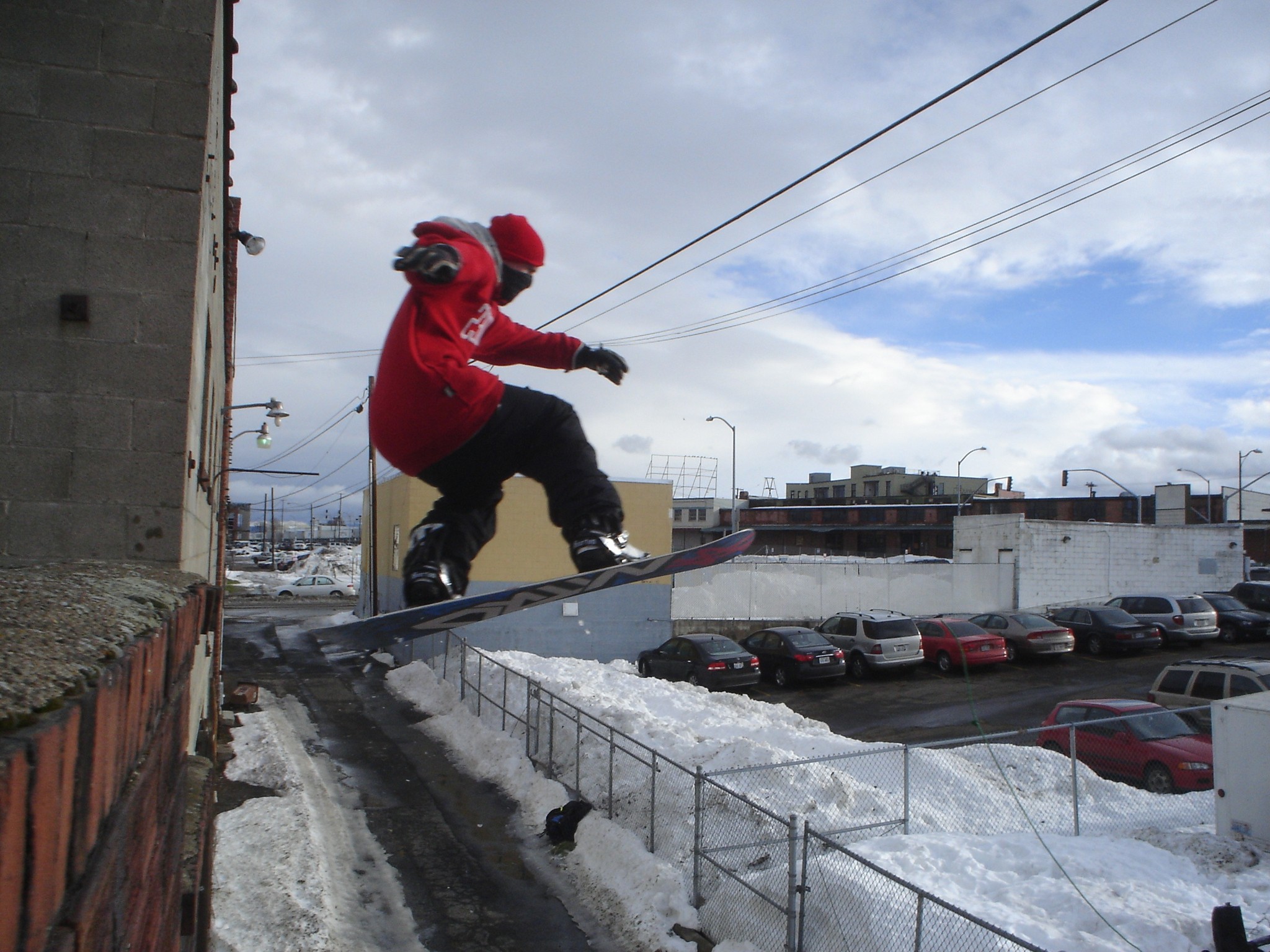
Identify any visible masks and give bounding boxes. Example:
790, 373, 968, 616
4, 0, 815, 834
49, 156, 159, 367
501, 263, 533, 302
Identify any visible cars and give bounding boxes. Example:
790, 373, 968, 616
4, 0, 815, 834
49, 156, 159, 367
1200, 593, 1270, 643
1047, 604, 1163, 656
634, 633, 762, 694
269, 574, 356, 599
1034, 698, 1214, 795
1225, 562, 1270, 612
967, 611, 1076, 664
225, 540, 355, 567
915, 618, 1008, 672
737, 626, 846, 688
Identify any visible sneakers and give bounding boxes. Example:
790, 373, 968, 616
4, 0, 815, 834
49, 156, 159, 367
402, 522, 464, 607
561, 511, 651, 573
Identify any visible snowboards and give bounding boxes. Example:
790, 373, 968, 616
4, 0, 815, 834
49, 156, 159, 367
307, 529, 756, 650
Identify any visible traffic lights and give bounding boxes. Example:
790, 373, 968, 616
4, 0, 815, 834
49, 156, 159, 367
1062, 470, 1069, 486
1007, 477, 1013, 491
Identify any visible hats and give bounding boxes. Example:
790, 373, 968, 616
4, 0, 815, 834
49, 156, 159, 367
488, 213, 545, 266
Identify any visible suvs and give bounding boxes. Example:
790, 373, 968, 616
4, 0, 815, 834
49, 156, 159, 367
810, 608, 924, 677
1104, 594, 1220, 648
1146, 654, 1270, 734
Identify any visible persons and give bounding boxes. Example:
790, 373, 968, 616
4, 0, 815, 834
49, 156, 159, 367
368, 215, 651, 610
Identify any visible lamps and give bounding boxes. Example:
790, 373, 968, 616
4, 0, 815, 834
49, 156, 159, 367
221, 398, 290, 426
238, 231, 266, 256
230, 422, 272, 448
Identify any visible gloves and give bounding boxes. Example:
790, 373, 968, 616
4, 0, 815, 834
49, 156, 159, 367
571, 341, 629, 385
393, 242, 461, 284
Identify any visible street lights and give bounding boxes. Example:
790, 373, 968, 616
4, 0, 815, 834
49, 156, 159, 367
706, 416, 739, 534
1177, 468, 1211, 524
1239, 449, 1263, 522
958, 446, 987, 516
